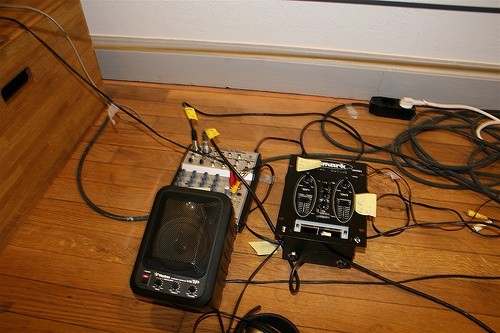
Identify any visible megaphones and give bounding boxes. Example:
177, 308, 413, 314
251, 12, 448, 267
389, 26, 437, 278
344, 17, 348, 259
130, 183, 237, 315
